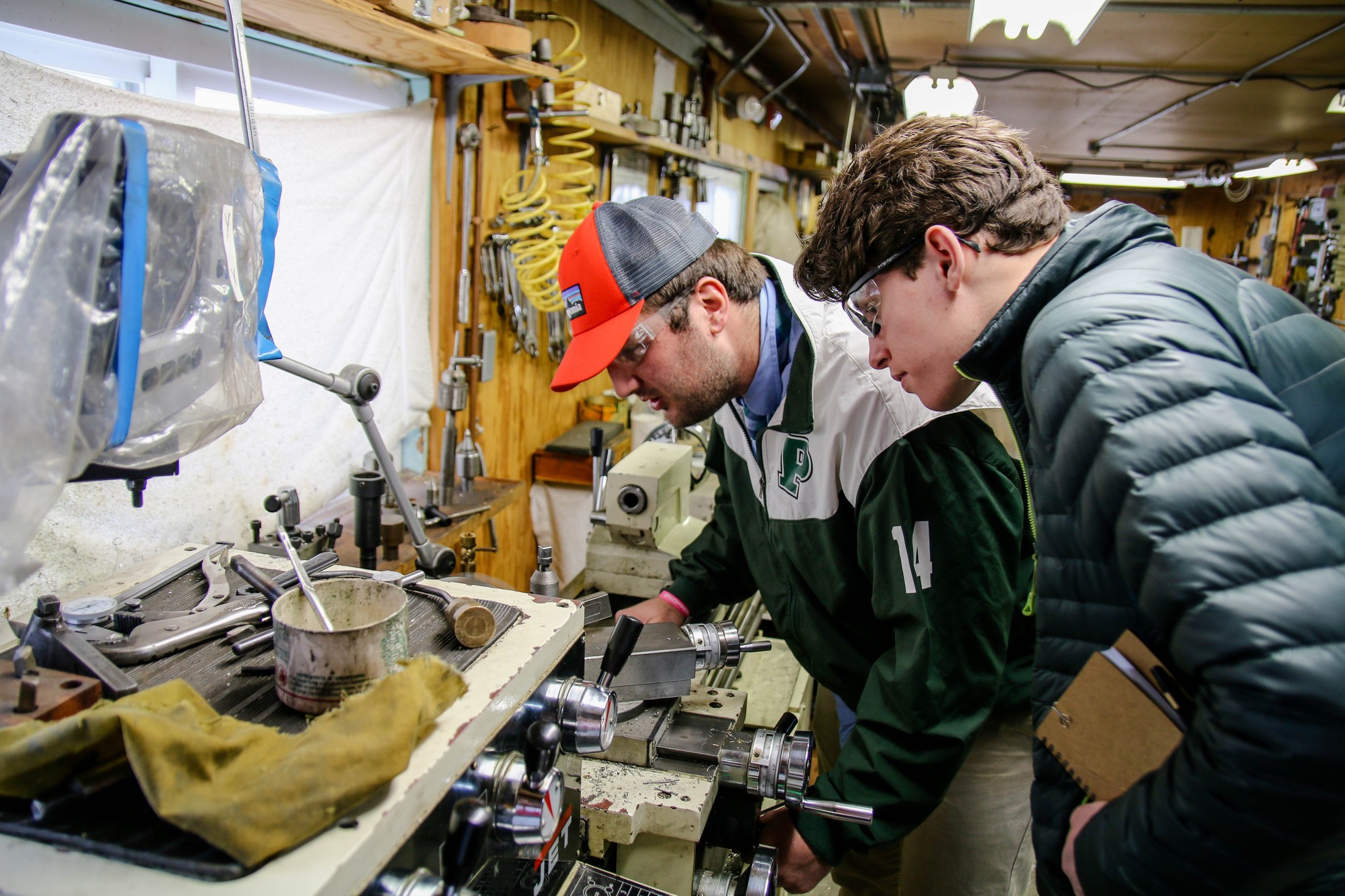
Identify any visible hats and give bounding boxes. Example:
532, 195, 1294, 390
551, 197, 718, 393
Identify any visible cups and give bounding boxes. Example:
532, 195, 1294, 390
271, 578, 408, 714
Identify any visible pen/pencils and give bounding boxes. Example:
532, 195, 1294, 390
1150, 665, 1197, 727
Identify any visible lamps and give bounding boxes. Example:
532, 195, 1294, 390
902, 45, 979, 120
1167, 152, 1319, 187
1325, 83, 1345, 114
967, 0, 1110, 45
1058, 166, 1187, 190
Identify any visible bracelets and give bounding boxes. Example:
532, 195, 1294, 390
658, 590, 689, 617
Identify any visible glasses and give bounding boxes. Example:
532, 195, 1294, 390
841, 236, 982, 337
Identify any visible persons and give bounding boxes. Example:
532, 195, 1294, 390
547, 194, 1046, 896
792, 112, 1345, 896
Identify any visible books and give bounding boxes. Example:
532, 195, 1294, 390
1035, 627, 1195, 803
